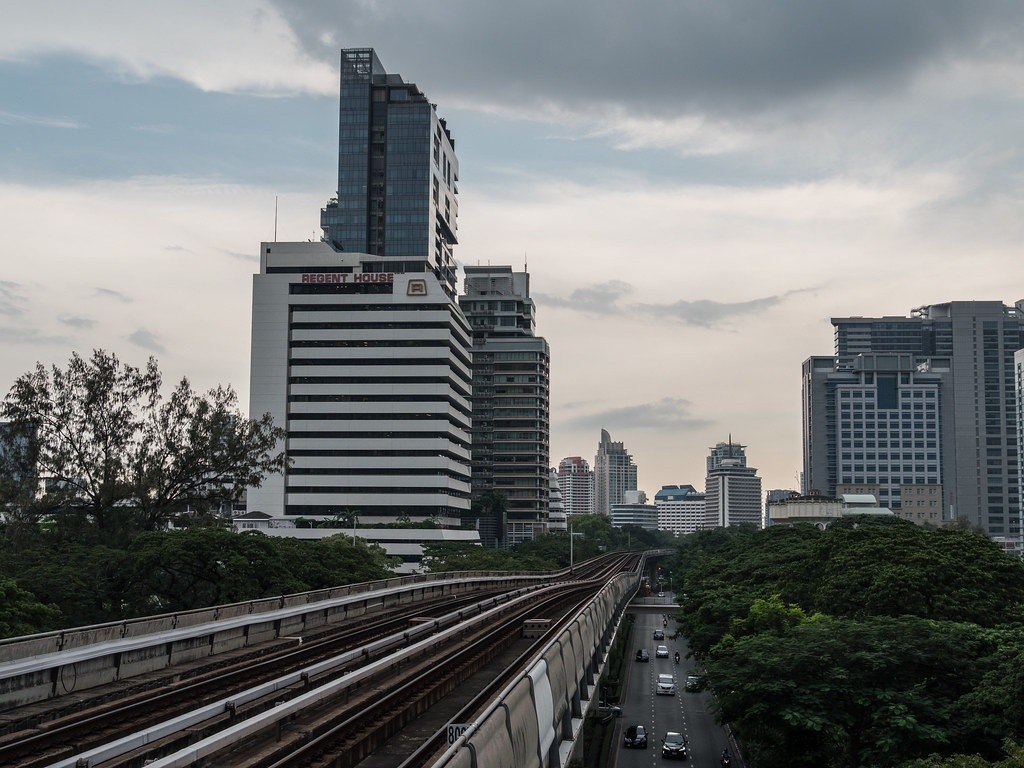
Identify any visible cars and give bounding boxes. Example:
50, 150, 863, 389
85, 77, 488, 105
661, 731, 689, 760
656, 645, 669, 658
654, 630, 664, 640
685, 676, 700, 692
624, 725, 649, 749
635, 649, 650, 661
657, 591, 665, 597
655, 673, 677, 695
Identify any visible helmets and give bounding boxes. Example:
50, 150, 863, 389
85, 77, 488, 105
724, 748, 728, 751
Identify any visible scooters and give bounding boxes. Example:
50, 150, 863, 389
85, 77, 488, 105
675, 656, 679, 663
720, 753, 732, 768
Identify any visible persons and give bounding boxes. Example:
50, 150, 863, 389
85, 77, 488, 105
663, 618, 667, 623
674, 651, 680, 660
722, 748, 731, 766
